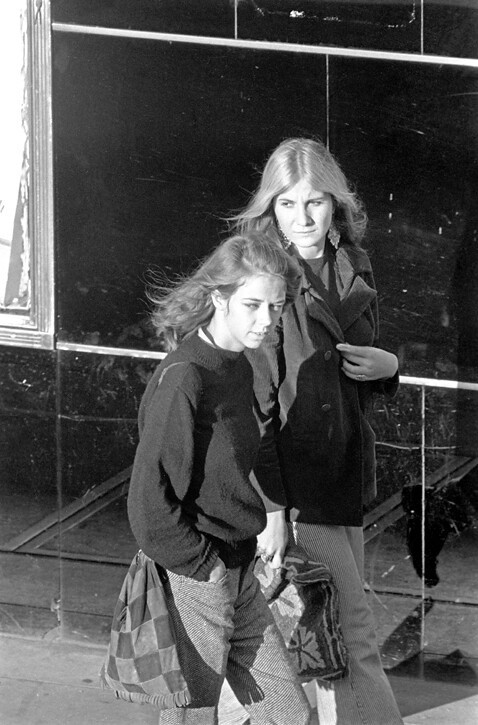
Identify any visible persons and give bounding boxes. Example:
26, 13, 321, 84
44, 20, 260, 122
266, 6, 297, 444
126, 137, 405, 725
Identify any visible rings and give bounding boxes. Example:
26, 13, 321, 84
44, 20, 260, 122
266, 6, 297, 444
356, 375, 366, 382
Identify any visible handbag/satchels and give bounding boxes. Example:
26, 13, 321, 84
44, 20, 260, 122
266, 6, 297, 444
97, 551, 193, 708
252, 545, 350, 683
